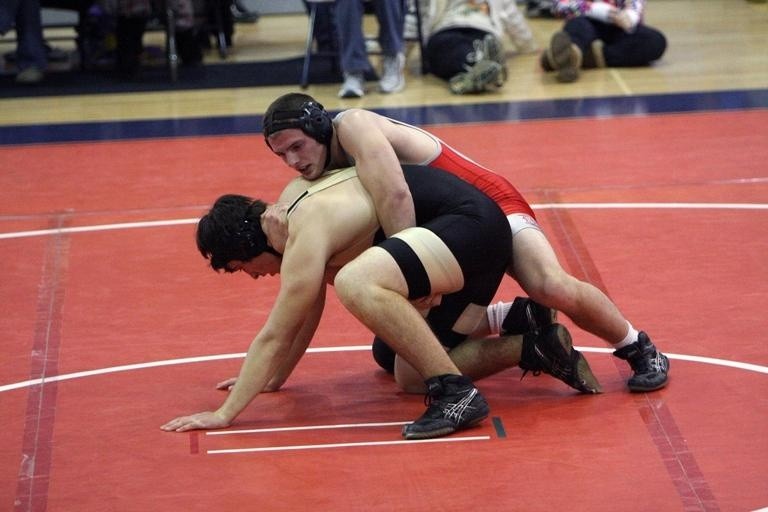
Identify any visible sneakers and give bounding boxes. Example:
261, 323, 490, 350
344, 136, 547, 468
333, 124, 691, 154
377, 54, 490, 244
403, 373, 489, 439
517, 323, 602, 393
611, 331, 669, 393
500, 296, 554, 337
339, 32, 581, 98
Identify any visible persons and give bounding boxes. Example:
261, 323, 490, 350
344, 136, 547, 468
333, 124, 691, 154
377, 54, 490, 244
2, 1, 669, 95
161, 165, 600, 440
262, 96, 669, 392
